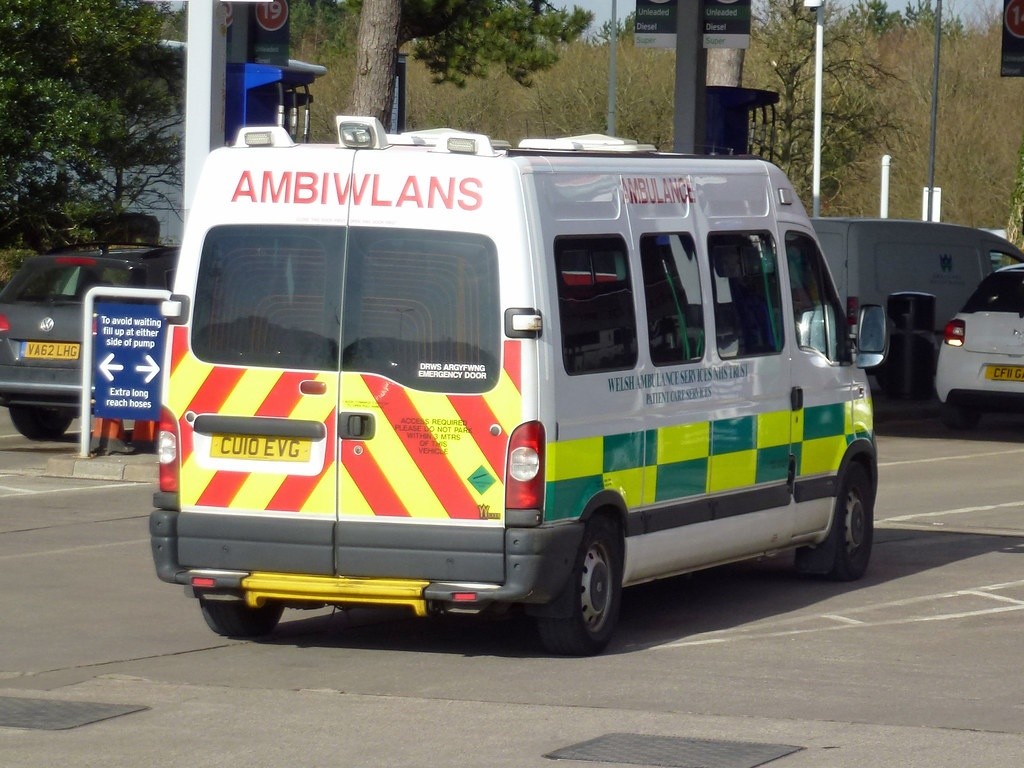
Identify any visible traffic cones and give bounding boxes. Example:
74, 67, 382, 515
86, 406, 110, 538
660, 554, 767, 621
88, 418, 135, 455
130, 420, 157, 452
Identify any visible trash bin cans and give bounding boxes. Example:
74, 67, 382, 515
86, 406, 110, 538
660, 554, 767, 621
886, 291, 936, 401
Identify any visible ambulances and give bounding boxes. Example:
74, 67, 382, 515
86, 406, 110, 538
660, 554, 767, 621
150, 115, 890, 659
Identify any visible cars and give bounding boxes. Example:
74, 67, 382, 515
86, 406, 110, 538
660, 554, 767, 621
933, 264, 1024, 430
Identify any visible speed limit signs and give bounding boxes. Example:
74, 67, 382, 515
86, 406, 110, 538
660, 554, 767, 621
255, 0, 288, 31
1004, 0, 1024, 38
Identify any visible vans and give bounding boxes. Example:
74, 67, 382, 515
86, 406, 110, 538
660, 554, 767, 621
809, 217, 1024, 400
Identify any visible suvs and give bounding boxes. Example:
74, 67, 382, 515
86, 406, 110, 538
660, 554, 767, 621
0, 242, 180, 441
